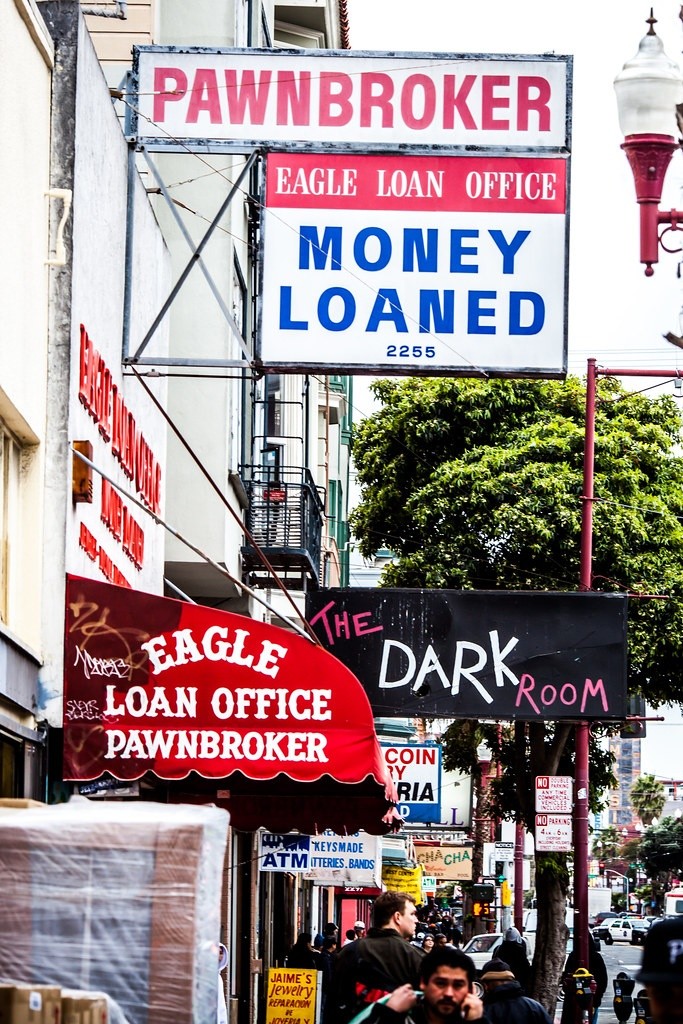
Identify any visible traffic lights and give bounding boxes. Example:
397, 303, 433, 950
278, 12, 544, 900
496, 861, 504, 886
471, 902, 491, 917
629, 864, 643, 869
599, 862, 605, 875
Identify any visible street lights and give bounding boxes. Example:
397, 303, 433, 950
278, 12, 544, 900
603, 868, 629, 911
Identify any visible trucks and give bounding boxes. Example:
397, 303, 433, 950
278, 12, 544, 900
587, 887, 612, 926
530, 898, 570, 909
664, 889, 683, 919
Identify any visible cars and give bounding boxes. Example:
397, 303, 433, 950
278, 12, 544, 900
462, 932, 536, 970
591, 911, 664, 945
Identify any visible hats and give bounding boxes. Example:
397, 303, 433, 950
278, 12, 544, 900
417, 932, 425, 939
354, 921, 365, 930
506, 927, 522, 944
325, 923, 339, 932
480, 958, 515, 979
635, 918, 683, 984
314, 934, 324, 946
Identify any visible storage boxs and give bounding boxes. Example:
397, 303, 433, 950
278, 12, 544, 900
0, 984, 107, 1024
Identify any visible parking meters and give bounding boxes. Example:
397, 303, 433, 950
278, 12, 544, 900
613, 972, 635, 1024
633, 989, 652, 1024
572, 968, 597, 1024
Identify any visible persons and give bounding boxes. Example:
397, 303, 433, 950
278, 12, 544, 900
560, 931, 608, 1024
287, 892, 554, 1024
633, 917, 683, 1024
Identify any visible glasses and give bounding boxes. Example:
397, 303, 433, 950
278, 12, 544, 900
220, 951, 224, 955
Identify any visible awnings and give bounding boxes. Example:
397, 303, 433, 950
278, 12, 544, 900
63, 572, 404, 836
